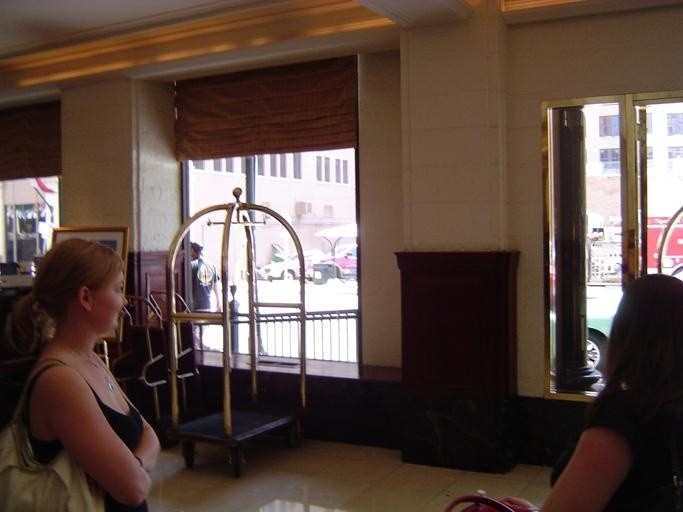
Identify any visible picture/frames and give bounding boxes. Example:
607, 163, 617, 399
51, 228, 129, 345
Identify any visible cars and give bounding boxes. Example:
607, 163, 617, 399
549, 270, 683, 382
254, 245, 356, 284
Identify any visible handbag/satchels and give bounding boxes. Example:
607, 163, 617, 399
0, 424, 104, 512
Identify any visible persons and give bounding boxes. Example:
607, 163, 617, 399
12, 237, 161, 511
187, 242, 220, 313
501, 274, 683, 512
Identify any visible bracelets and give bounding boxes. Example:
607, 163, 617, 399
135, 455, 144, 467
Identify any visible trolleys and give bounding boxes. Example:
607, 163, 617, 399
164, 189, 310, 476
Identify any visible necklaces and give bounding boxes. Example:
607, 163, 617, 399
68, 341, 115, 393
